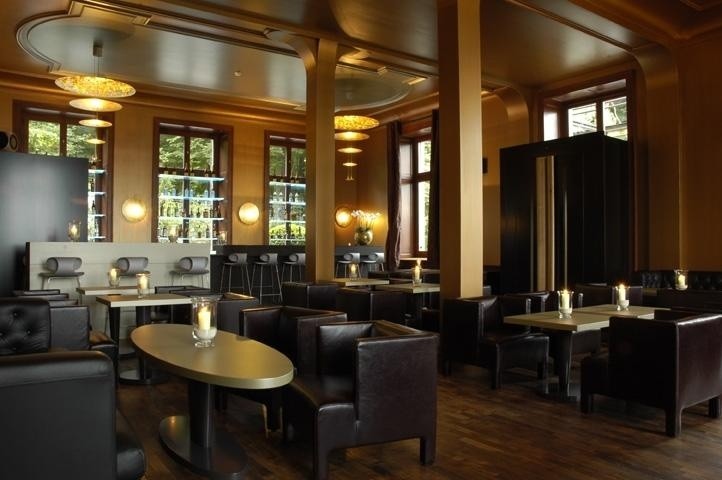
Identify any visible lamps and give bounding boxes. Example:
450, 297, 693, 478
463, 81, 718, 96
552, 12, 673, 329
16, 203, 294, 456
334, 114, 380, 181
239, 202, 259, 225
55, 73, 136, 145
335, 206, 352, 229
121, 197, 147, 223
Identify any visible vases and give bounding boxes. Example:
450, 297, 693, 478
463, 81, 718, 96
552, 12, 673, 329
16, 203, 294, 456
353, 228, 373, 246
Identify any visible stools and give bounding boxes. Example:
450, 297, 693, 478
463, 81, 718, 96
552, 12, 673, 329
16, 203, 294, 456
362, 261, 384, 274
170, 270, 209, 287
251, 262, 283, 305
118, 270, 152, 288
335, 261, 360, 278
285, 261, 306, 282
220, 262, 253, 296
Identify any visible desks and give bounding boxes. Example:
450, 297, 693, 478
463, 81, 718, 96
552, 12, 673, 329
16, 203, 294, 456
38, 272, 83, 306
571, 304, 671, 320
503, 309, 610, 403
130, 323, 295, 480
95, 293, 193, 385
374, 283, 441, 330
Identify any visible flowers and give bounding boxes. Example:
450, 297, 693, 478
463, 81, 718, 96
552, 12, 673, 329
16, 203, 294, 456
351, 209, 382, 232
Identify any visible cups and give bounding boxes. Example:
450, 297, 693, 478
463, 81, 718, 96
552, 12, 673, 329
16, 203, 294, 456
348, 263, 359, 282
219, 230, 228, 246
673, 269, 690, 291
411, 267, 423, 287
613, 285, 631, 311
136, 273, 150, 299
191, 297, 218, 348
65, 219, 81, 242
557, 290, 574, 320
108, 269, 121, 288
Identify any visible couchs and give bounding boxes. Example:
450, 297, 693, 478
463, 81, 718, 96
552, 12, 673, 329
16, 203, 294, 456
289, 317, 442, 479
614, 269, 722, 313
0, 290, 146, 480
444, 283, 613, 390
579, 308, 721, 438
154, 284, 259, 330
240, 306, 348, 376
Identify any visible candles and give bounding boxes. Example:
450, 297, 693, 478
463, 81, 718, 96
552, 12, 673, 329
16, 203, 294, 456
676, 271, 686, 290
561, 289, 570, 309
139, 273, 148, 292
618, 283, 626, 302
351, 263, 356, 277
71, 222, 77, 238
197, 302, 211, 332
111, 268, 117, 283
413, 266, 420, 282
